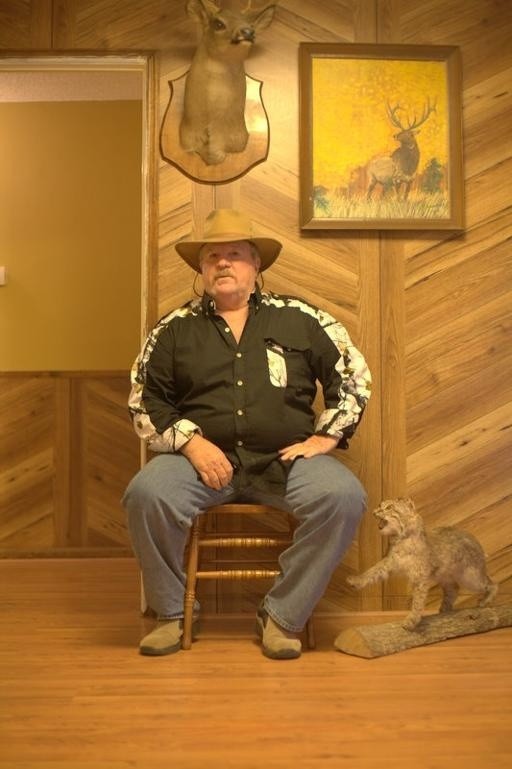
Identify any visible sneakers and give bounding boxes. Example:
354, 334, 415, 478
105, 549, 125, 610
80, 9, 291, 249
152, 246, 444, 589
139, 613, 203, 655
254, 605, 303, 660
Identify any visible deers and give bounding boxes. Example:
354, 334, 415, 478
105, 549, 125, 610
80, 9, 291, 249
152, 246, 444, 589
365, 96, 438, 209
180, 0, 277, 166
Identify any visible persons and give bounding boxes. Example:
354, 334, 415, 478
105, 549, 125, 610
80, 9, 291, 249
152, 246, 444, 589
118, 207, 377, 659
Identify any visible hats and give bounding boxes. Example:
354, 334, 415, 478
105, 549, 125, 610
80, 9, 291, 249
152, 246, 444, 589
174, 207, 283, 275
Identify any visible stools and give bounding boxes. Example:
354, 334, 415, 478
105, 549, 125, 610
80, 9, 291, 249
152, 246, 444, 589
181, 501, 320, 650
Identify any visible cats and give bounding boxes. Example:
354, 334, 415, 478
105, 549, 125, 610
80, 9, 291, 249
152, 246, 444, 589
344, 496, 498, 632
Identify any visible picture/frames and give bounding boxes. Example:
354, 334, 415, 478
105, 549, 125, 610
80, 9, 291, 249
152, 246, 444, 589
297, 39, 470, 234
